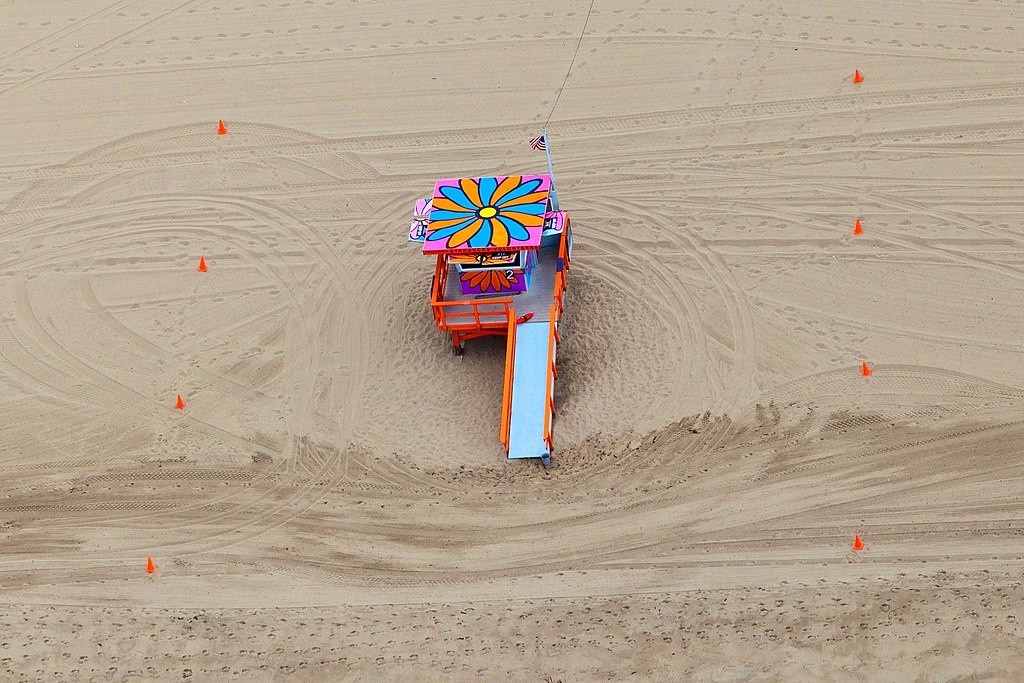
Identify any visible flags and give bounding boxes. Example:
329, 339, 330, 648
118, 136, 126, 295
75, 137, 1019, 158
529, 135, 546, 151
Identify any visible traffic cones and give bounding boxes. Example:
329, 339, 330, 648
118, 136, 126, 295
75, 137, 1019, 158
197, 256, 208, 272
852, 533, 864, 551
853, 220, 864, 235
145, 556, 155, 573
862, 361, 872, 377
216, 119, 230, 135
853, 69, 865, 83
176, 393, 186, 410
